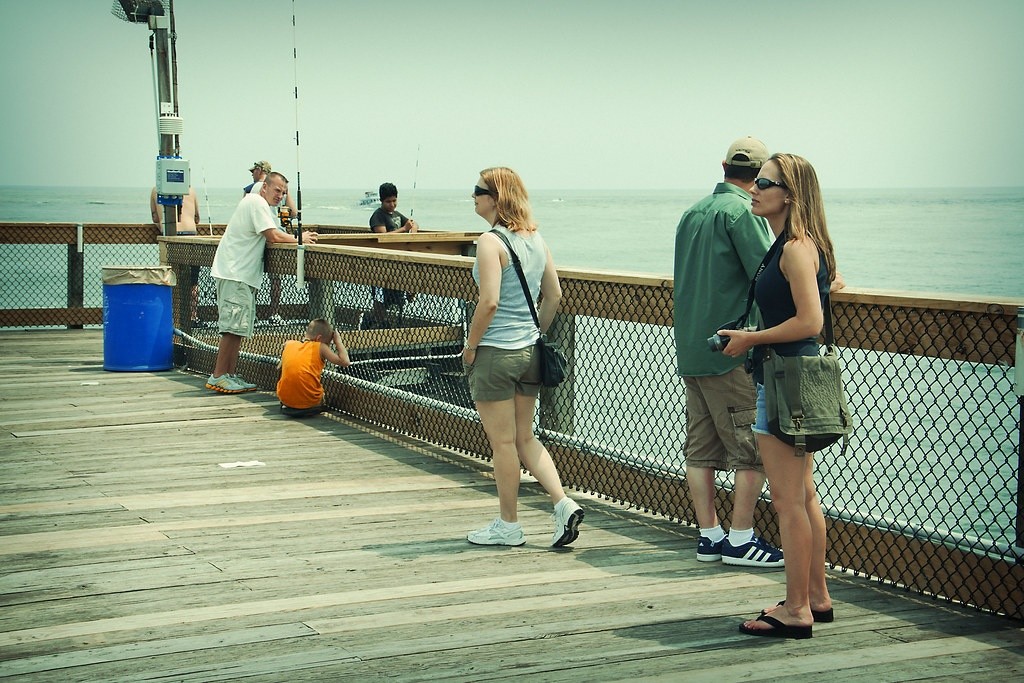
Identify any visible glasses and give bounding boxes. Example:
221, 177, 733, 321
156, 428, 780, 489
754, 177, 787, 190
475, 185, 497, 196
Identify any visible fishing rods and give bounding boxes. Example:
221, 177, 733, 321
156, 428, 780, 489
409, 143, 420, 233
200, 164, 213, 236
292, 0, 305, 288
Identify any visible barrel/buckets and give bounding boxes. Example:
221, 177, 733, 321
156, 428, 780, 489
100, 266, 176, 372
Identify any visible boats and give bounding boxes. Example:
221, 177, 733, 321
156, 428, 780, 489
359, 191, 382, 209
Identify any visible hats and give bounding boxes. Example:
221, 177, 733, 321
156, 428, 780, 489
725, 135, 770, 168
249, 160, 271, 175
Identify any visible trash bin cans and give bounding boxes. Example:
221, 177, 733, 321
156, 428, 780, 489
103, 266, 173, 370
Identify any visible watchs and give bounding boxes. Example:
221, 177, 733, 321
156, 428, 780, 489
463, 341, 477, 350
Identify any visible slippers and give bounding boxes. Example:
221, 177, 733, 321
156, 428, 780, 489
739, 614, 812, 639
761, 599, 834, 622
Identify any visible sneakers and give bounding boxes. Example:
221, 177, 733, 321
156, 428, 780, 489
696, 528, 729, 562
466, 516, 527, 547
205, 374, 245, 394
228, 374, 258, 391
550, 498, 586, 548
721, 531, 785, 568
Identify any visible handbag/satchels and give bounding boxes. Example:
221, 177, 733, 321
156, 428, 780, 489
536, 337, 568, 389
762, 346, 853, 458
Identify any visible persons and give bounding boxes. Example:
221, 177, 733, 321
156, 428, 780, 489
204, 172, 318, 395
739, 153, 836, 640
276, 318, 350, 416
358, 182, 419, 331
242, 161, 296, 325
461, 168, 585, 549
674, 138, 845, 568
149, 180, 208, 328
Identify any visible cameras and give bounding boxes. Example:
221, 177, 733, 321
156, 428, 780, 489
707, 321, 742, 352
